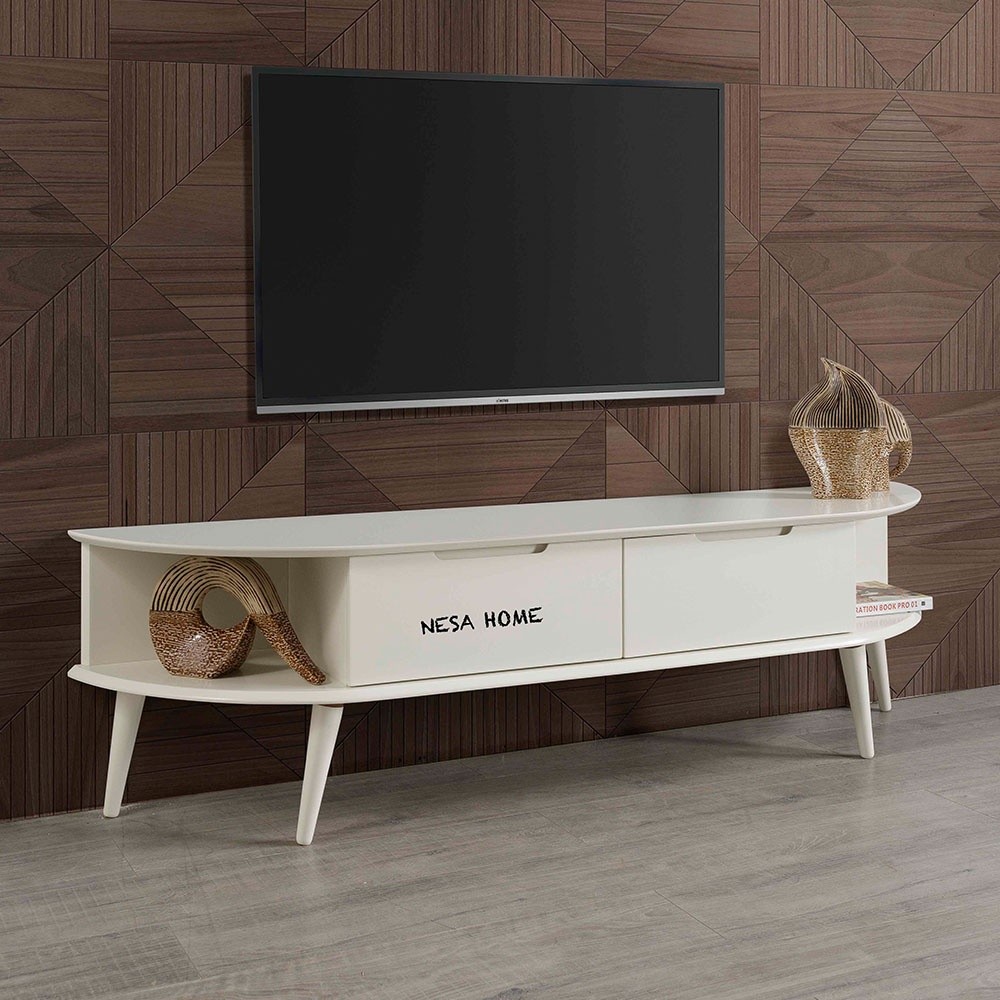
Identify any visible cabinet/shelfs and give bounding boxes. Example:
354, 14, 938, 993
67, 480, 925, 846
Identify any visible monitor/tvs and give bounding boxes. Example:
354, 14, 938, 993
252, 67, 726, 414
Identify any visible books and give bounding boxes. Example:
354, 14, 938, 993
855, 580, 933, 617
